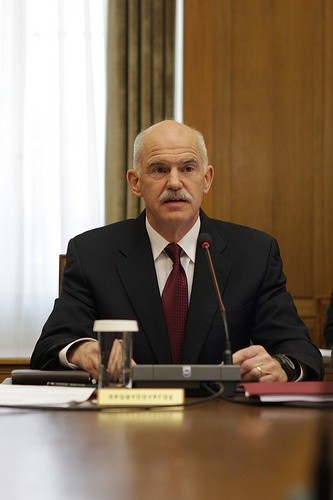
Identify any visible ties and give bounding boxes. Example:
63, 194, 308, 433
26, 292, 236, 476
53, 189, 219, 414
162, 244, 188, 364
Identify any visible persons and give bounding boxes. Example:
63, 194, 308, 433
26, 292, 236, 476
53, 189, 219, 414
30, 119, 325, 383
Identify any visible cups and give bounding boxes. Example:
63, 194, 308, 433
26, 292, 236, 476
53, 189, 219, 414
93, 320, 139, 389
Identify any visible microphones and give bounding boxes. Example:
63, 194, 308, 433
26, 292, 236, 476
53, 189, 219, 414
131, 234, 241, 400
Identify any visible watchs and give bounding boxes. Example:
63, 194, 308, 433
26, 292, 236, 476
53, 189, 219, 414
273, 353, 297, 382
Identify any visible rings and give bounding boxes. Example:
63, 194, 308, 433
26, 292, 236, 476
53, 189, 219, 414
256, 366, 263, 376
